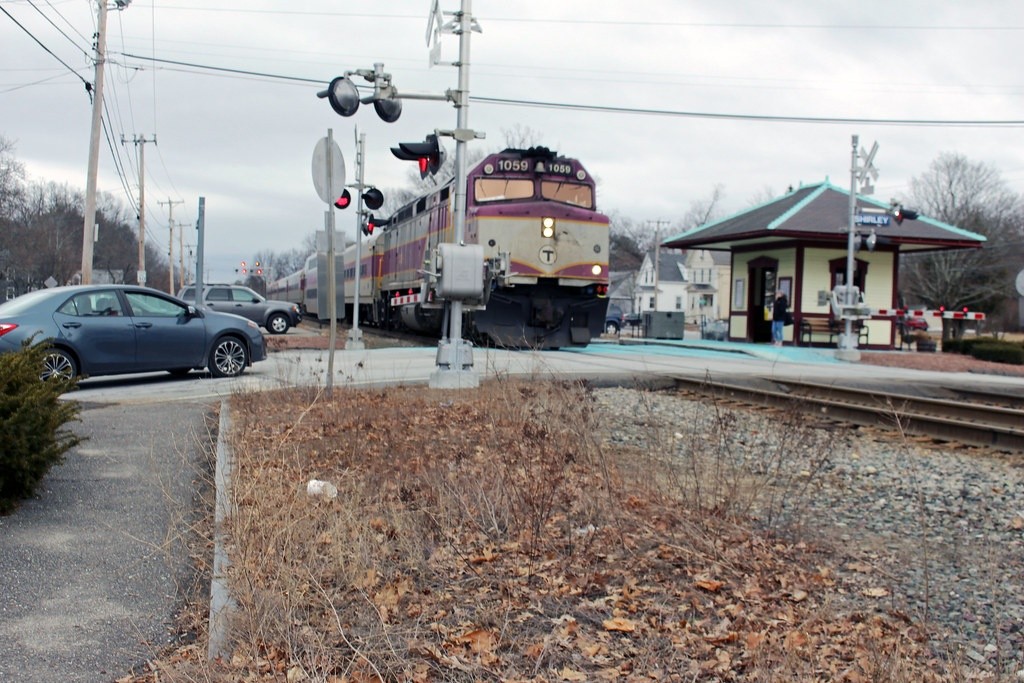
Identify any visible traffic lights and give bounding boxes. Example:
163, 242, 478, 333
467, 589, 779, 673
361, 215, 376, 237
333, 189, 352, 210
390, 134, 448, 180
893, 205, 919, 225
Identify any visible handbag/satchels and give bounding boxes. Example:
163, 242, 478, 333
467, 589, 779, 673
784, 312, 794, 326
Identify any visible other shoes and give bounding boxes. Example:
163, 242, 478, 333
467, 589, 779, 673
773, 341, 782, 346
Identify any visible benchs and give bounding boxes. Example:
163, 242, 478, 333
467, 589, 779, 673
800, 317, 869, 345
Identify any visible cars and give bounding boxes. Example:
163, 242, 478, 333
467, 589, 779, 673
175, 283, 303, 335
1, 284, 268, 392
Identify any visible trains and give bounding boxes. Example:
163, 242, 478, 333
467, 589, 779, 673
266, 146, 610, 350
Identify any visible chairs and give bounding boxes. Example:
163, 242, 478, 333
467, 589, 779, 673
96, 298, 112, 310
77, 297, 91, 313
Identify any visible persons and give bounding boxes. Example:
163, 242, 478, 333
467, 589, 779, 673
772, 290, 786, 346
